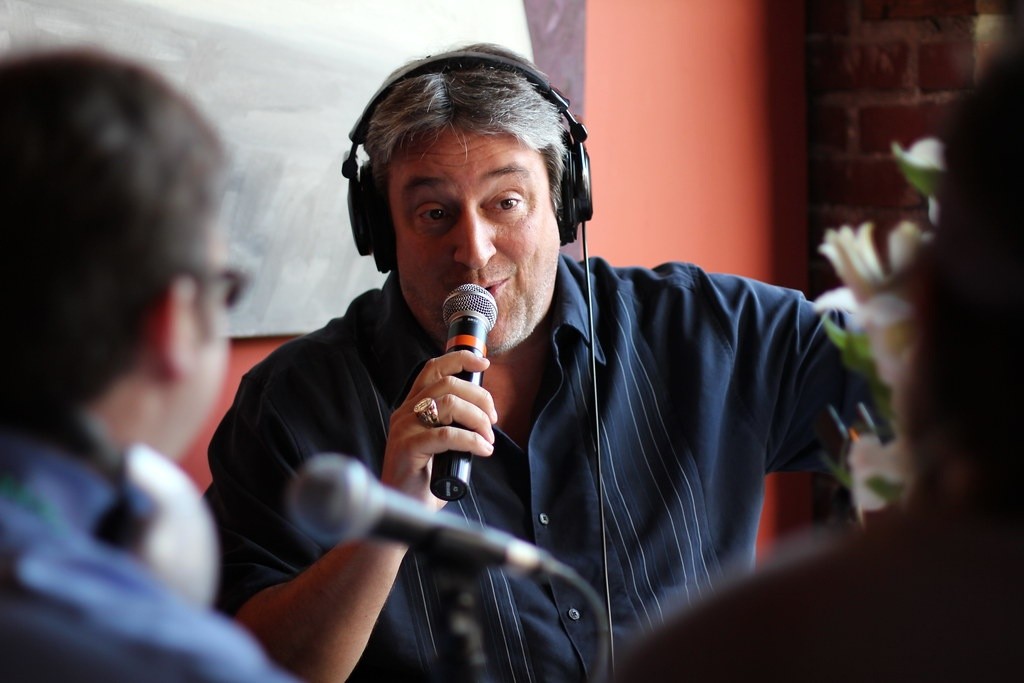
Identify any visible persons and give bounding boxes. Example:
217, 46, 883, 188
201, 43, 897, 682
0, 49, 301, 682
612, 10, 1023, 683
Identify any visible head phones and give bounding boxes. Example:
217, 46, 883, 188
343, 51, 593, 276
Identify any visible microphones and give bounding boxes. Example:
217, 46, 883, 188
431, 281, 498, 503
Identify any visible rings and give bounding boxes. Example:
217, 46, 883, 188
414, 398, 439, 428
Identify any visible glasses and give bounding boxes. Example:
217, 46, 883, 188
173, 265, 249, 309
